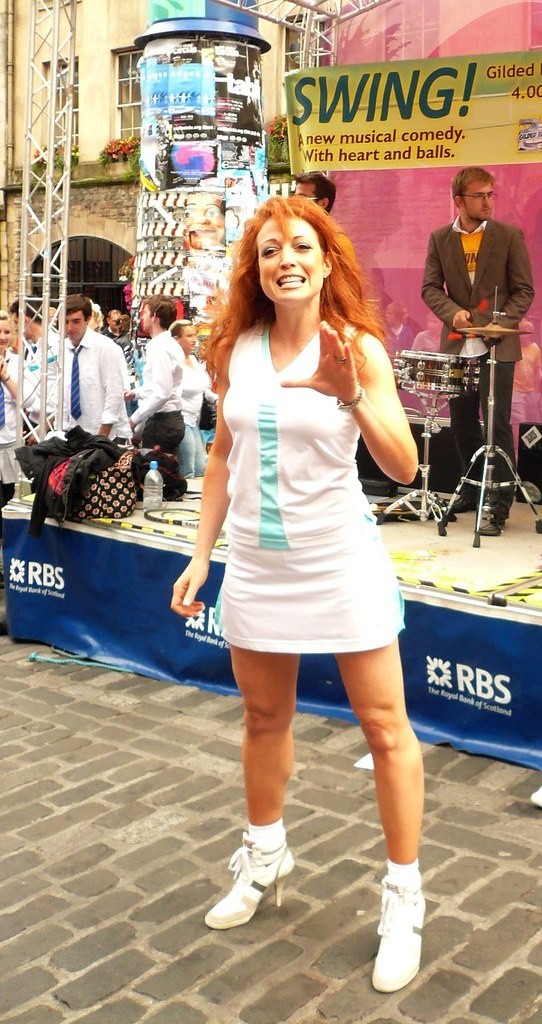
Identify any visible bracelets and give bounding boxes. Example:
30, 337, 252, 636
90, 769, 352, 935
336, 384, 364, 412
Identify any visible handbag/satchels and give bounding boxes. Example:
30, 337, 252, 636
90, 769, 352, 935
69, 452, 137, 518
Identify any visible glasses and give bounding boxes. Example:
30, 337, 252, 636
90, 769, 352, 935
460, 193, 497, 200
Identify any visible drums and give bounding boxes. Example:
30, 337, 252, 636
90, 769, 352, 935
397, 348, 480, 394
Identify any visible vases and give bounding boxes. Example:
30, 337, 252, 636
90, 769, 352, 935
54, 166, 79, 180
104, 162, 133, 176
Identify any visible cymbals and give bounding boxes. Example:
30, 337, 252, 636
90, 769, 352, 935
455, 322, 533, 338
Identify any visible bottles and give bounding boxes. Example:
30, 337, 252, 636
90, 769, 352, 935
143, 461, 164, 513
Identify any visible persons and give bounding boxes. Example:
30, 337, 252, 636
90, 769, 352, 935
0, 294, 217, 508
169, 196, 426, 994
378, 298, 542, 451
420, 167, 535, 537
290, 171, 357, 265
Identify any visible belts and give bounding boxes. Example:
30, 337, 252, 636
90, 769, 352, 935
150, 411, 181, 418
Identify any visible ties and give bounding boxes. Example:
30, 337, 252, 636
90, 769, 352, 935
71, 346, 83, 420
0, 378, 6, 430
32, 346, 37, 360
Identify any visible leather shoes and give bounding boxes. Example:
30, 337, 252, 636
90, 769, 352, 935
451, 497, 477, 512
489, 503, 509, 525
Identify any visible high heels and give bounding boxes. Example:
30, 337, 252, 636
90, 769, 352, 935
205, 832, 294, 930
372, 875, 426, 993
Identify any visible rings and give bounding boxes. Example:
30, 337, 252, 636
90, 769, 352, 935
336, 357, 347, 363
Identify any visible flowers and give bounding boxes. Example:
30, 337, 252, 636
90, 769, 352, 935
98, 135, 141, 171
32, 141, 80, 175
